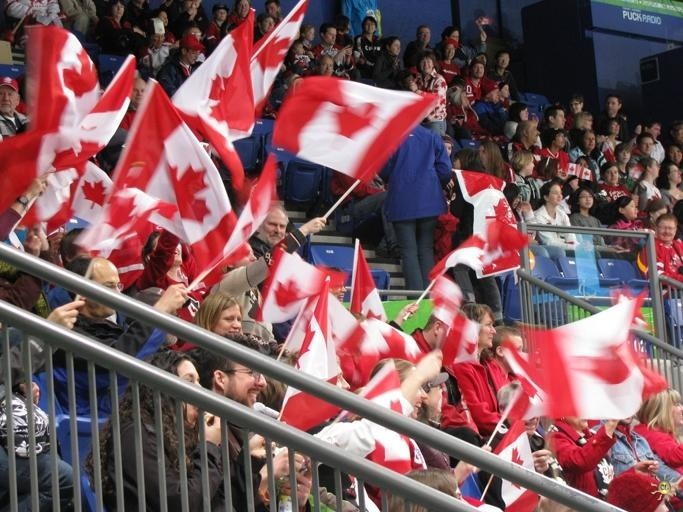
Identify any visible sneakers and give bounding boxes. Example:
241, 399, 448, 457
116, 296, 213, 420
375, 248, 388, 257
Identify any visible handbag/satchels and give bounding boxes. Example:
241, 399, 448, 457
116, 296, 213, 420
0, 388, 54, 455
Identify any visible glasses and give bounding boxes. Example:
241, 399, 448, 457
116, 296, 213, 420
320, 64, 332, 67
548, 192, 561, 195
577, 195, 592, 198
221, 368, 254, 375
104, 283, 123, 292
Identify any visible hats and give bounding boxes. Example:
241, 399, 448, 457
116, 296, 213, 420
0, 76, 19, 90
212, 3, 229, 12
548, 128, 563, 144
147, 17, 165, 33
180, 35, 203, 50
63, 216, 88, 234
64, 257, 104, 301
441, 37, 458, 49
481, 75, 498, 97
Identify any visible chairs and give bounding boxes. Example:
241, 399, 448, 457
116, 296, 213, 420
0, 64, 24, 79
341, 269, 389, 301
56, 415, 110, 512
526, 93, 549, 110
521, 101, 544, 122
252, 119, 275, 139
311, 245, 354, 272
220, 132, 260, 183
500, 257, 683, 358
281, 161, 326, 217
260, 132, 293, 186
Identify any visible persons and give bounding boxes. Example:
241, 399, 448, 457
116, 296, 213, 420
0, 2, 682, 511
